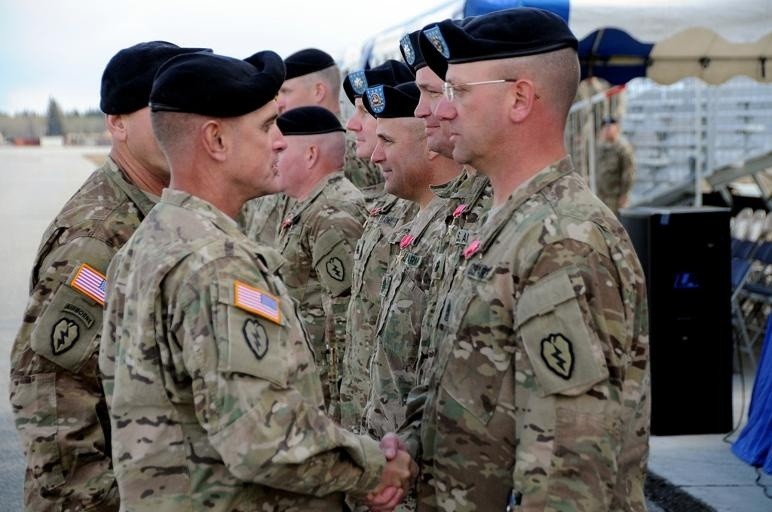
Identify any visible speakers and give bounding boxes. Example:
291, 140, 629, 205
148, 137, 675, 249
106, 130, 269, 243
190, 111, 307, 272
619, 207, 733, 435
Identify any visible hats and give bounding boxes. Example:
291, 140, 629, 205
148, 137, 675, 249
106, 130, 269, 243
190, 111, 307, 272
282, 46, 337, 82
361, 79, 424, 120
98, 38, 214, 117
277, 105, 348, 138
150, 48, 287, 118
395, 18, 463, 76
342, 59, 406, 108
419, 6, 581, 66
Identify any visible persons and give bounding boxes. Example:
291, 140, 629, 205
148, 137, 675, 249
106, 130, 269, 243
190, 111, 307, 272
8, 39, 216, 511
96, 44, 412, 511
383, 21, 495, 512
418, 7, 655, 510
594, 115, 636, 214
237, 48, 386, 423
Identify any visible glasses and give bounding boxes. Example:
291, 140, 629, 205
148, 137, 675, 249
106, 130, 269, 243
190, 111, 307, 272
441, 77, 542, 104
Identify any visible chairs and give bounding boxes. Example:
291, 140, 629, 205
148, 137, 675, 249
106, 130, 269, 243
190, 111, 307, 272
729, 209, 772, 372
